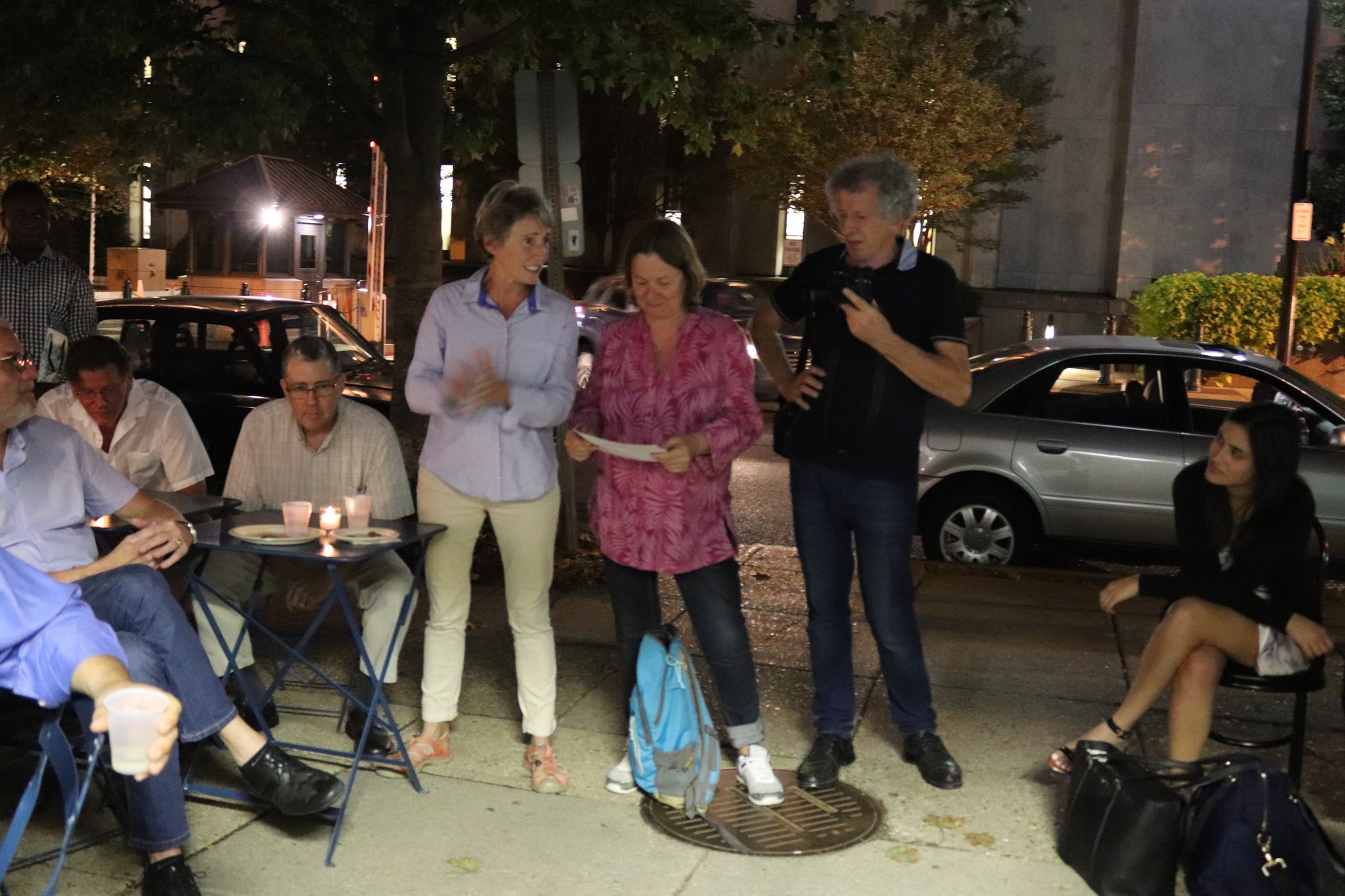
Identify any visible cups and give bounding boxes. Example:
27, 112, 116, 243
101, 687, 170, 774
318, 506, 342, 531
280, 501, 312, 537
343, 494, 373, 529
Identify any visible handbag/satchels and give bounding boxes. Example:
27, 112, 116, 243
1182, 751, 1345, 896
36, 327, 69, 384
772, 394, 805, 457
1057, 739, 1265, 896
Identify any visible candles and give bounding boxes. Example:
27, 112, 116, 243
320, 506, 342, 531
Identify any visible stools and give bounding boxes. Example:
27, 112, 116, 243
1207, 653, 1326, 795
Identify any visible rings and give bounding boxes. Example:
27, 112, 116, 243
174, 539, 184, 546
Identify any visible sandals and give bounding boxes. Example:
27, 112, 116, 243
1049, 719, 1133, 775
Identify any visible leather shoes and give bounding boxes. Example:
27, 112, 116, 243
902, 733, 962, 790
215, 687, 280, 747
797, 732, 855, 789
344, 702, 393, 756
238, 740, 346, 815
140, 852, 207, 896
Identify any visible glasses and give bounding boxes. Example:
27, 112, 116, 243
0, 350, 34, 369
75, 380, 123, 401
284, 374, 340, 400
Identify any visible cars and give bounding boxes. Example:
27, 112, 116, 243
574, 272, 819, 402
92, 293, 399, 497
912, 333, 1345, 583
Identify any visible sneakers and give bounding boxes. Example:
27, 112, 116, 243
605, 747, 638, 795
522, 740, 567, 795
376, 726, 452, 779
736, 743, 786, 806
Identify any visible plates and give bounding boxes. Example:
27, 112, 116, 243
228, 524, 327, 545
329, 528, 400, 542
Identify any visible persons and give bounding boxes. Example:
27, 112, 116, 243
568, 217, 781, 805
0, 544, 182, 782
0, 323, 346, 896
1049, 399, 1334, 791
29, 331, 216, 636
0, 175, 102, 406
750, 149, 977, 790
194, 335, 421, 758
405, 181, 580, 796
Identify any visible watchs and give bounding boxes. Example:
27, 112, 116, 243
175, 519, 199, 545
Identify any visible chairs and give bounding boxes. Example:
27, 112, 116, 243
0, 691, 123, 896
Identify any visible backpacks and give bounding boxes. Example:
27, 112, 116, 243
627, 622, 753, 853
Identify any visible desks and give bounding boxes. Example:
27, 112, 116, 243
87, 490, 447, 866
